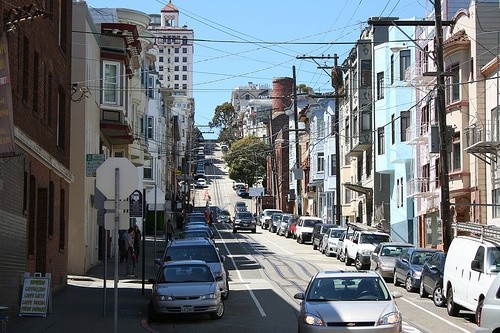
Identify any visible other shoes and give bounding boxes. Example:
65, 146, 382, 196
126, 274, 135, 278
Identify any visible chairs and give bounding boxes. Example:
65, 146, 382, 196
165, 269, 178, 280
314, 279, 341, 299
355, 279, 381, 298
191, 268, 206, 280
383, 248, 390, 254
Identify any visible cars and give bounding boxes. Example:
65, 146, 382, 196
154, 237, 229, 301
150, 259, 225, 322
294, 271, 403, 333
259, 209, 299, 239
476, 272, 500, 333
311, 223, 338, 253
322, 228, 347, 257
296, 216, 323, 244
392, 249, 442, 293
370, 242, 415, 279
419, 253, 447, 307
180, 201, 257, 239
336, 231, 353, 261
232, 180, 247, 197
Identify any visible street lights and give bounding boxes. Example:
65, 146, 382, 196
188, 146, 204, 205
240, 158, 258, 215
275, 139, 303, 217
310, 103, 341, 225
390, 44, 451, 252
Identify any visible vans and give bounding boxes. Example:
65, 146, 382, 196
442, 222, 500, 317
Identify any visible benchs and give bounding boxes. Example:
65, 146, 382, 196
335, 288, 358, 298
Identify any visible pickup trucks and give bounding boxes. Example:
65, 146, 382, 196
341, 231, 401, 270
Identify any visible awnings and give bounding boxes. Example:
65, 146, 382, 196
463, 141, 500, 165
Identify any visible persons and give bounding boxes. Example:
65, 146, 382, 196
118, 227, 142, 279
209, 211, 213, 225
163, 219, 175, 242
